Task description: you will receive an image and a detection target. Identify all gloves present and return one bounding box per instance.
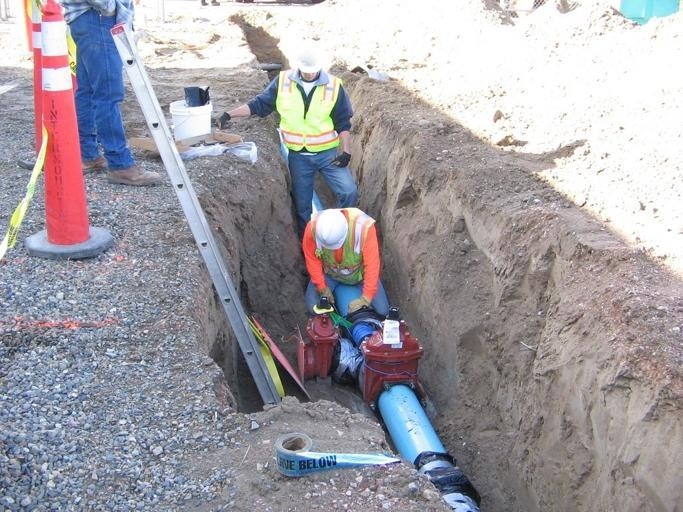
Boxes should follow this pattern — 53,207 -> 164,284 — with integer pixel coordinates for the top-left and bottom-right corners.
334,152 -> 352,171
216,112 -> 231,131
347,297 -> 370,313
318,287 -> 337,303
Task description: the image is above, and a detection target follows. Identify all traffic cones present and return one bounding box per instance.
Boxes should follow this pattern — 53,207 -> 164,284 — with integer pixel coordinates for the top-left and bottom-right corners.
15,0 -> 45,167
10,0 -> 118,263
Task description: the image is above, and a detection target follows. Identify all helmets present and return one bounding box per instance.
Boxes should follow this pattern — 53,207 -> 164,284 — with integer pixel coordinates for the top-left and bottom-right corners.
296,46 -> 326,74
317,208 -> 348,250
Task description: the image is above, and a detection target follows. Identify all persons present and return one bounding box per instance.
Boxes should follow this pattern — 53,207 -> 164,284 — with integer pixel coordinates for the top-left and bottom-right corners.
60,0 -> 161,188
216,43 -> 358,239
302,207 -> 390,317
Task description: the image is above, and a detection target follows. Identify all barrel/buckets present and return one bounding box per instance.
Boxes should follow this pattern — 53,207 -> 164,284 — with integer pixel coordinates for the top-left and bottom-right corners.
169,98 -> 213,142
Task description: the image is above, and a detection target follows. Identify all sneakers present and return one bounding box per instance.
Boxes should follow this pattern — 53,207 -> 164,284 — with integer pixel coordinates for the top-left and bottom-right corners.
108,164 -> 161,187
81,154 -> 110,172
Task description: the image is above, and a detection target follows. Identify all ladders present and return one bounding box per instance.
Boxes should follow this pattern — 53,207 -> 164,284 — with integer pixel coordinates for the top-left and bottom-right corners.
110,21 -> 282,407
